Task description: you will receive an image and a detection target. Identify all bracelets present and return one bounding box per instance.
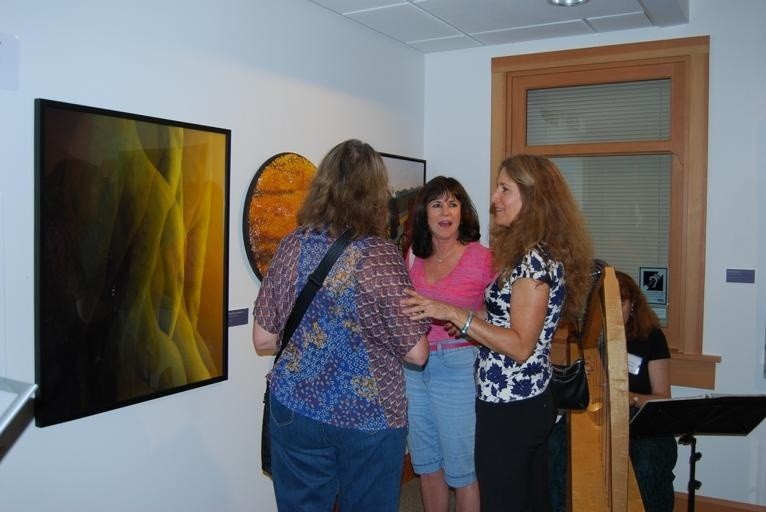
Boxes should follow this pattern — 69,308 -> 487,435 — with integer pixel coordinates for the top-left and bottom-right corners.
630,394 -> 639,409
461,311 -> 473,334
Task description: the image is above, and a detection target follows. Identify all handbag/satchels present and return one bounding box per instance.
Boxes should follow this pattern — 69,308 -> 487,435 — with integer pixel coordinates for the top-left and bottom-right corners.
260,380 -> 271,473
550,358 -> 589,410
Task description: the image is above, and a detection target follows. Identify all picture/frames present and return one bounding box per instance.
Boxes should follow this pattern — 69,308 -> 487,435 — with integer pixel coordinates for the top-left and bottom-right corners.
243,151 -> 318,282
380,153 -> 426,198
34,98 -> 231,428
639,267 -> 667,305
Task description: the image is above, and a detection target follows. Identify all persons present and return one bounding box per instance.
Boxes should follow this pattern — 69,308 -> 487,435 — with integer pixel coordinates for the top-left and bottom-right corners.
599,271 -> 678,512
253,140 -> 433,511
399,154 -> 593,512
403,176 -> 495,512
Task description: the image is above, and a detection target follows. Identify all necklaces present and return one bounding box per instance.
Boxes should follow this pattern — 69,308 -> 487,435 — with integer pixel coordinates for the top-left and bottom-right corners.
435,245 -> 454,263
